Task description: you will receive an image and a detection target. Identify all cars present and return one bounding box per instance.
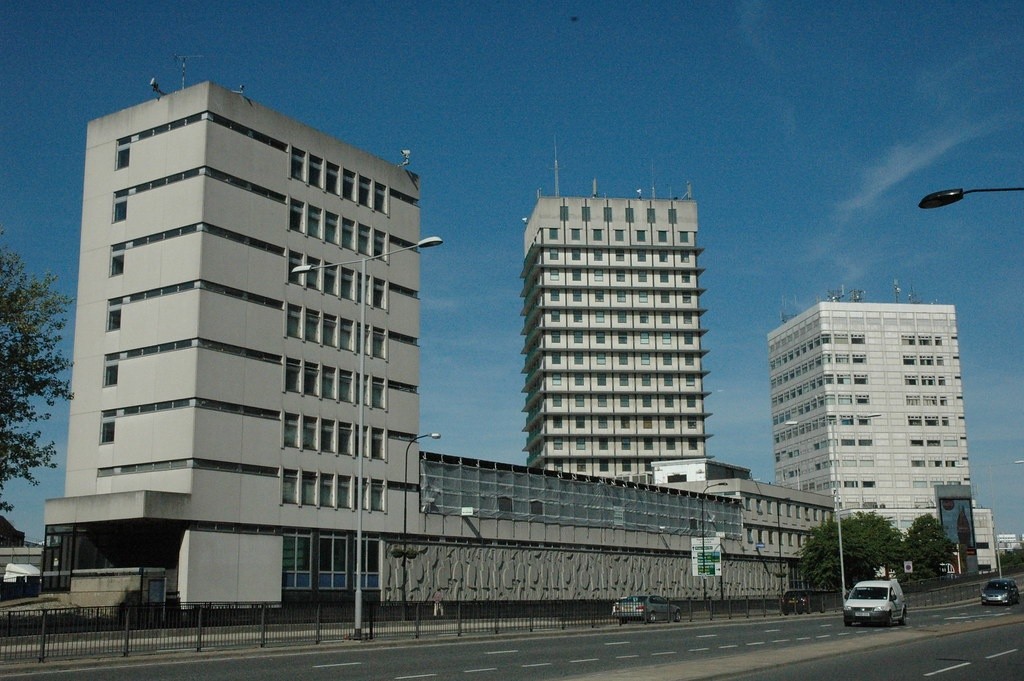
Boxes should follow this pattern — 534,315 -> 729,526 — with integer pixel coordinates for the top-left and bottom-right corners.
843,578 -> 907,627
612,594 -> 682,623
981,578 -> 1020,606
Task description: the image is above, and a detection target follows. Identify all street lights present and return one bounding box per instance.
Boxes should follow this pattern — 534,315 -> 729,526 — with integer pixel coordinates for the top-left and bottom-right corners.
402,433 -> 441,620
777,496 -> 791,596
292,235 -> 441,639
701,481 -> 729,612
954,460 -> 1024,578
785,413 -> 882,608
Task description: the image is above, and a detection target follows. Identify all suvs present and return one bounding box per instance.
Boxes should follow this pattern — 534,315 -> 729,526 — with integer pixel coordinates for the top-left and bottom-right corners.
781,591 -> 825,616
